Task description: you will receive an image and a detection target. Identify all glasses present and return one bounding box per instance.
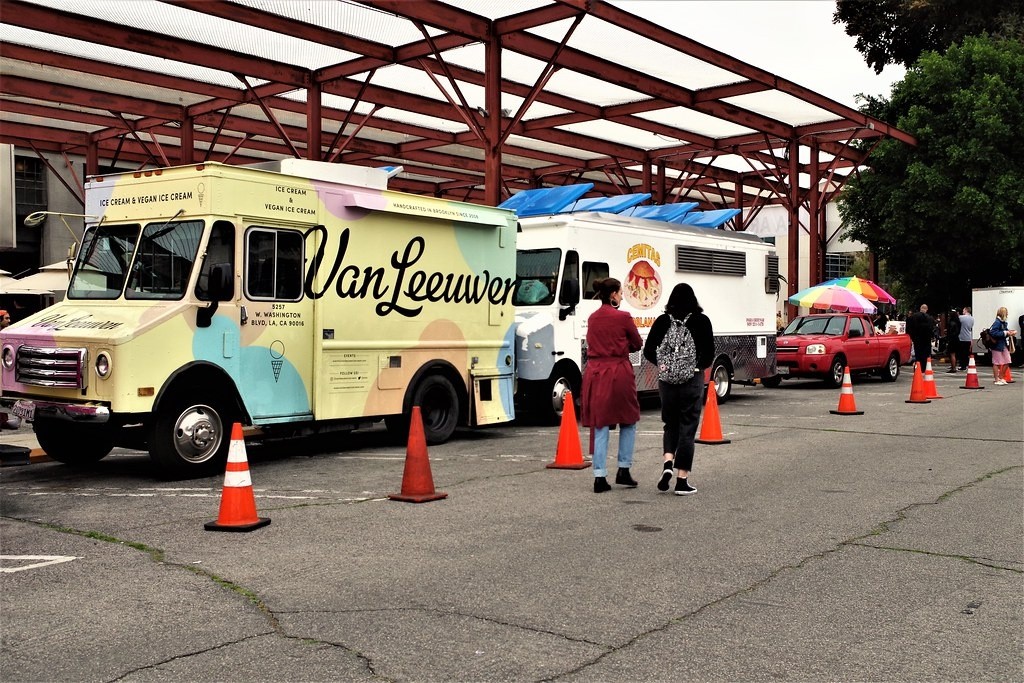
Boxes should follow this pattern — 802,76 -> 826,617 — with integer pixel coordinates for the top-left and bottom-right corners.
5,317 -> 10,319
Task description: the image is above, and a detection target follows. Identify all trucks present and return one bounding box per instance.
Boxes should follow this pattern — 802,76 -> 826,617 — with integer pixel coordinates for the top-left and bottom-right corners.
971,285 -> 1024,368
498,182 -> 781,425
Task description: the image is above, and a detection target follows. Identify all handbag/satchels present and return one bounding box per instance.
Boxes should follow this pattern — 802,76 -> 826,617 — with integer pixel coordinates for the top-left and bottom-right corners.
1009,335 -> 1015,354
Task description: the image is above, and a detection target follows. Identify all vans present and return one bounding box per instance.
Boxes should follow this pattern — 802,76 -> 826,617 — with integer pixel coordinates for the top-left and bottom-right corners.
0,152 -> 519,479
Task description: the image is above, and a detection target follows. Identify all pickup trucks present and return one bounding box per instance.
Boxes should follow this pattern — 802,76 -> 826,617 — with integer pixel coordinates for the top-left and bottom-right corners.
761,312 -> 913,389
780,304 -> 781,305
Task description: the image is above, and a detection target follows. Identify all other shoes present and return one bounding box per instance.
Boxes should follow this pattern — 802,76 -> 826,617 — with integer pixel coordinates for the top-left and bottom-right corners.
615,467 -> 638,486
946,370 -> 956,373
593,477 -> 611,493
958,367 -> 966,370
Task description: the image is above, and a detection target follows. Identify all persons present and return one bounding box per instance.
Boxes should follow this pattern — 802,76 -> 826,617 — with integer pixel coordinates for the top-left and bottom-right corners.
581,277 -> 644,493
911,304 -> 935,372
0,310 -> 11,331
643,282 -> 716,496
872,309 -> 912,331
989,307 -> 1015,386
947,306 -> 974,374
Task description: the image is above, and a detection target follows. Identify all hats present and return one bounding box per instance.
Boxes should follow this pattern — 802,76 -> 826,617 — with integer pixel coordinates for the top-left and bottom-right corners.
0,310 -> 7,316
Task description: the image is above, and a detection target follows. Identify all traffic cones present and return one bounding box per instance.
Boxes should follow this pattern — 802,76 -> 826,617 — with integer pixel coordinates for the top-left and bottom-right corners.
999,364 -> 1017,383
959,355 -> 985,389
545,391 -> 592,471
694,380 -> 732,445
204,421 -> 273,533
904,361 -> 932,403
923,357 -> 944,400
387,405 -> 449,503
828,365 -> 866,417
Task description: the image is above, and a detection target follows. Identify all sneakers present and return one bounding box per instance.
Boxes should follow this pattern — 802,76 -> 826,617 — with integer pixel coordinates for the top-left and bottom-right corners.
674,477 -> 697,495
995,379 -> 1008,386
657,460 -> 674,491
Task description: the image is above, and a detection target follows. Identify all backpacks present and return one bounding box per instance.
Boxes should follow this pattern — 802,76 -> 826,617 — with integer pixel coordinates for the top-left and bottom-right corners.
656,311 -> 697,384
977,319 -> 997,351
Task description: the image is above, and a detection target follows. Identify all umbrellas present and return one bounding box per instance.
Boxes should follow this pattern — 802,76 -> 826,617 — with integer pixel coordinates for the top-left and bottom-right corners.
789,284 -> 878,313
0,256 -> 107,295
815,274 -> 896,305
